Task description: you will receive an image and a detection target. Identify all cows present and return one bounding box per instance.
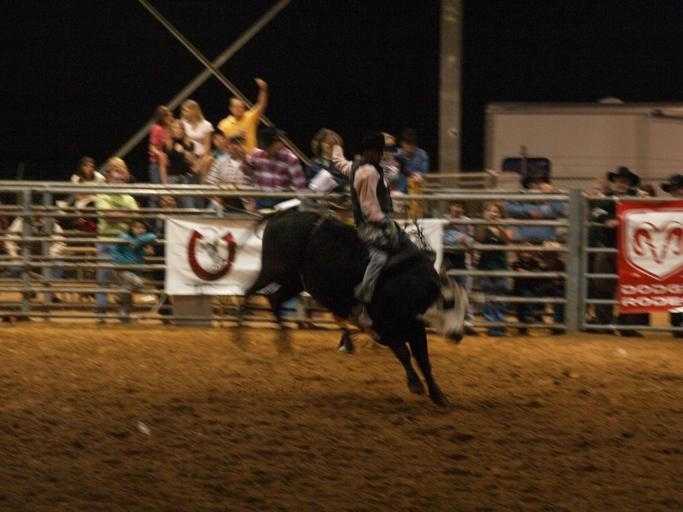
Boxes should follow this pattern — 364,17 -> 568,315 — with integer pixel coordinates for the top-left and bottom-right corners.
233,203 -> 471,410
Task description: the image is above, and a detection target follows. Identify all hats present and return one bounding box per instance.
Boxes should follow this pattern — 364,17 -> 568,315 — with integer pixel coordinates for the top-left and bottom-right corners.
355,129 -> 417,152
522,171 -> 548,188
662,175 -> 683,191
607,166 -> 640,186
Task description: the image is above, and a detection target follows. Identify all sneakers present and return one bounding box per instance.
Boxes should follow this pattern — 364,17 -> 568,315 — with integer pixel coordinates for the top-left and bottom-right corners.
348,305 -> 373,326
